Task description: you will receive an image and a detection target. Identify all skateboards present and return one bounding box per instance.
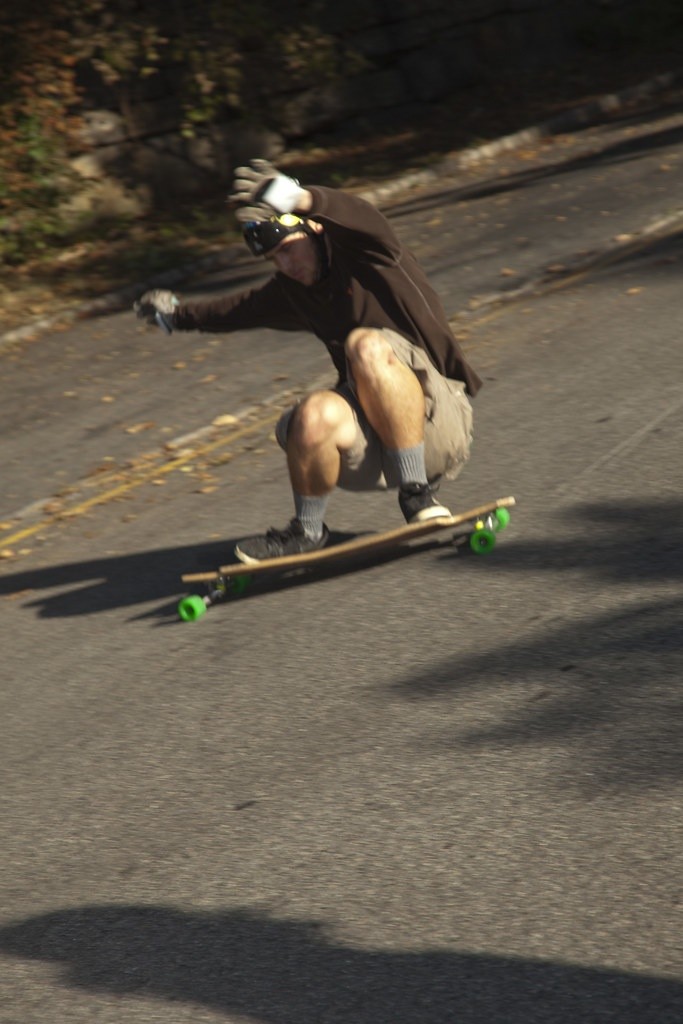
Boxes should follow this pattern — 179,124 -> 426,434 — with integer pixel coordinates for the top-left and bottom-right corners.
175,495 -> 517,619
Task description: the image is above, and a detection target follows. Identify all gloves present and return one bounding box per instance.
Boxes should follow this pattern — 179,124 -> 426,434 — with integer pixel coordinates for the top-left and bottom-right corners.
132,287 -> 178,332
228,158 -> 301,215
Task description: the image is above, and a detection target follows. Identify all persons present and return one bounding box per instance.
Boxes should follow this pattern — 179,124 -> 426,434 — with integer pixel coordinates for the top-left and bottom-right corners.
133,156 -> 481,564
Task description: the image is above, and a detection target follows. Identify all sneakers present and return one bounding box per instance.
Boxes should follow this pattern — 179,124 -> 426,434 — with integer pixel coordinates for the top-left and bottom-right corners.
397,483 -> 451,524
234,518 -> 330,568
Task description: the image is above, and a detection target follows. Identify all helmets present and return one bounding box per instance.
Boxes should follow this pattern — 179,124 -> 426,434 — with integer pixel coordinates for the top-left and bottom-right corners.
241,211 -> 308,256
